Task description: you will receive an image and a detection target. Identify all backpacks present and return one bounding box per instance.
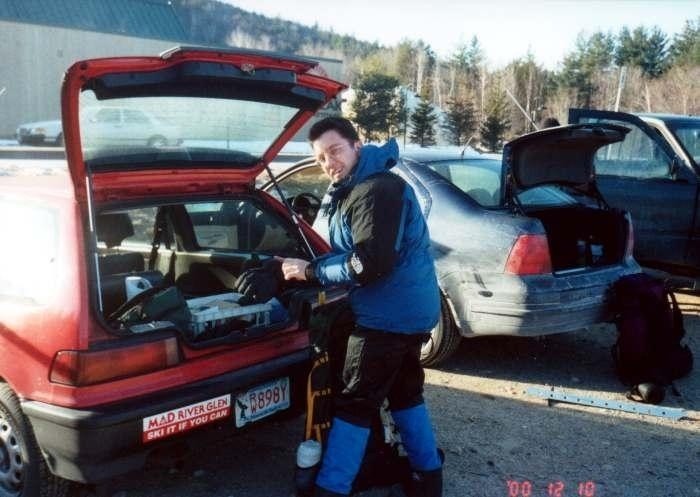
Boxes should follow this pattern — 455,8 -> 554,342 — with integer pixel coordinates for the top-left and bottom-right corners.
605,275 -> 693,383
295,350 -> 413,495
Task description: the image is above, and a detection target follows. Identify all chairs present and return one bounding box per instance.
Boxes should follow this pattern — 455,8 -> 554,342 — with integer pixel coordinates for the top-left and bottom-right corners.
95,214 -> 147,297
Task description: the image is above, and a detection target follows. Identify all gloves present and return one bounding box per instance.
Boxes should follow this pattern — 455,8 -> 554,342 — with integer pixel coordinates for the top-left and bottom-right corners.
234,262 -> 284,303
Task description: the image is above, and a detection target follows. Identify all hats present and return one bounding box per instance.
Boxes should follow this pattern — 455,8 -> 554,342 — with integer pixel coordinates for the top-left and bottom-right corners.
107,275 -> 161,315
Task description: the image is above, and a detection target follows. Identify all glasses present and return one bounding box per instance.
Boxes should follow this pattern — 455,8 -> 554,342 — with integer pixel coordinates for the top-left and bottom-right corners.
315,141 -> 351,168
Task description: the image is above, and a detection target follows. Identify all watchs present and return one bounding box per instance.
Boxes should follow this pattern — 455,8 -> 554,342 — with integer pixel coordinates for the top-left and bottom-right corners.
305,264 -> 317,282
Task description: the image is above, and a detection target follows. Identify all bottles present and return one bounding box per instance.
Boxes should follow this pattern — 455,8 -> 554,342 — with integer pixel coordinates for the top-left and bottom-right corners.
292,439 -> 324,492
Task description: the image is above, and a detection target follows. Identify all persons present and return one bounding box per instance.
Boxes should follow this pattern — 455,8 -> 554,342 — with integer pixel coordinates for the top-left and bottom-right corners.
273,118 -> 442,497
